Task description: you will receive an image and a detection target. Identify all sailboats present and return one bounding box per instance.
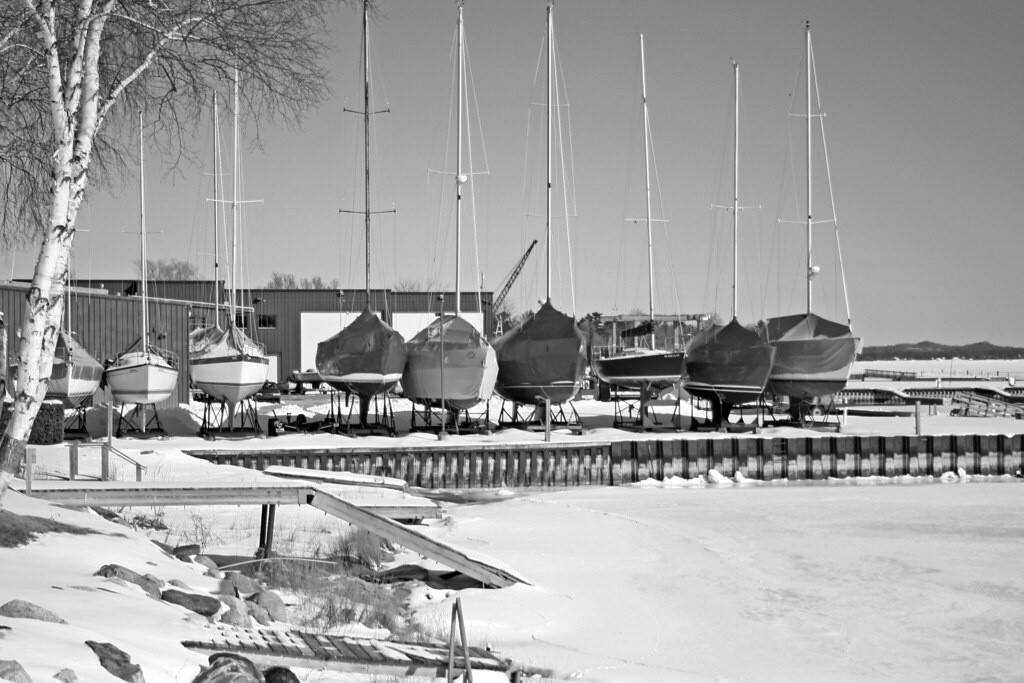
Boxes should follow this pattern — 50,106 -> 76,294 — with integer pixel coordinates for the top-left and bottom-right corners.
311,0 -> 410,392
750,16 -> 864,401
402,0 -> 502,411
99,107 -> 180,405
593,29 -> 688,386
183,57 -> 270,399
483,5 -> 587,407
676,58 -> 779,405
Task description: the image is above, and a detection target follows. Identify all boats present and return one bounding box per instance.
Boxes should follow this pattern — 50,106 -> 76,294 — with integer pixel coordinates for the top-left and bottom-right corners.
6,252 -> 104,405
